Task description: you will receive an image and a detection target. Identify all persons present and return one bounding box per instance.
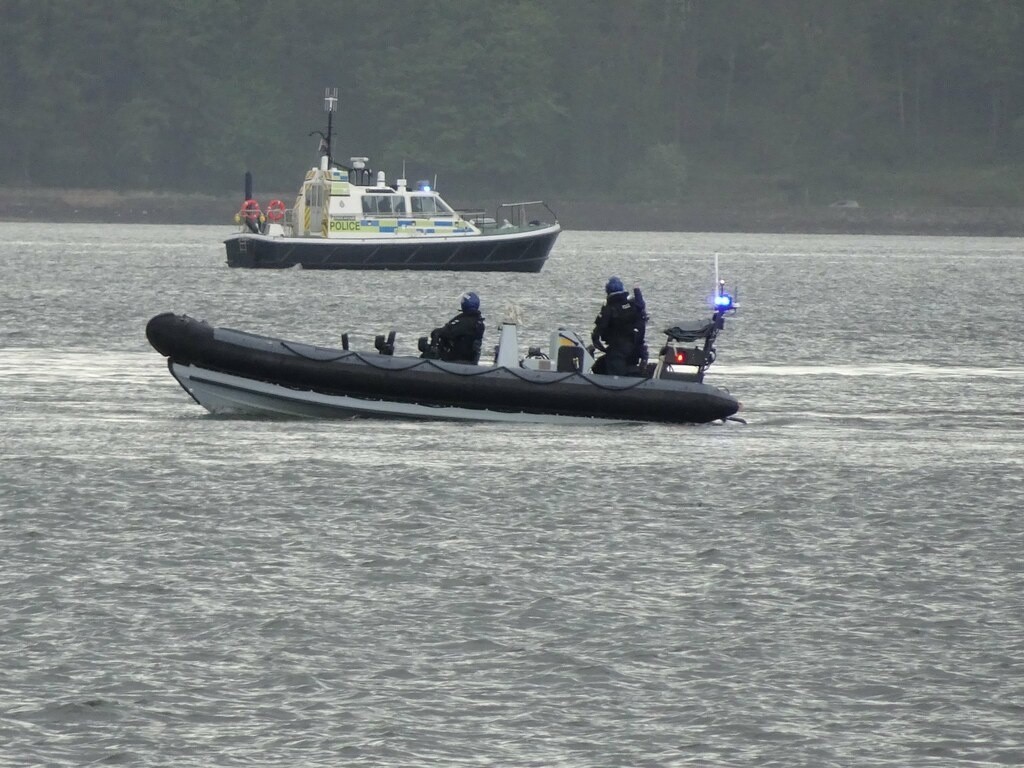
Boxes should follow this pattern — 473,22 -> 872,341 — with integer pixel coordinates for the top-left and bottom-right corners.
430,293 -> 486,364
362,197 -> 419,213
591,276 -> 648,376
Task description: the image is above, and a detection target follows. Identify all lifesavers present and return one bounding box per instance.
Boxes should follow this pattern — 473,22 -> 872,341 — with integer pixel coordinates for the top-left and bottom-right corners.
266,199 -> 287,221
240,199 -> 260,221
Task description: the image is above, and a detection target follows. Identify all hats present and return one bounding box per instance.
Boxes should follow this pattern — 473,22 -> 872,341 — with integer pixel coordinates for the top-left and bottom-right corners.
457,292 -> 480,312
605,276 -> 623,293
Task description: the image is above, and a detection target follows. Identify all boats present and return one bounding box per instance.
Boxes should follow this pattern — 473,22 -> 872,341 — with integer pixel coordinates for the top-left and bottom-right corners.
223,88 -> 562,275
146,253 -> 746,426
